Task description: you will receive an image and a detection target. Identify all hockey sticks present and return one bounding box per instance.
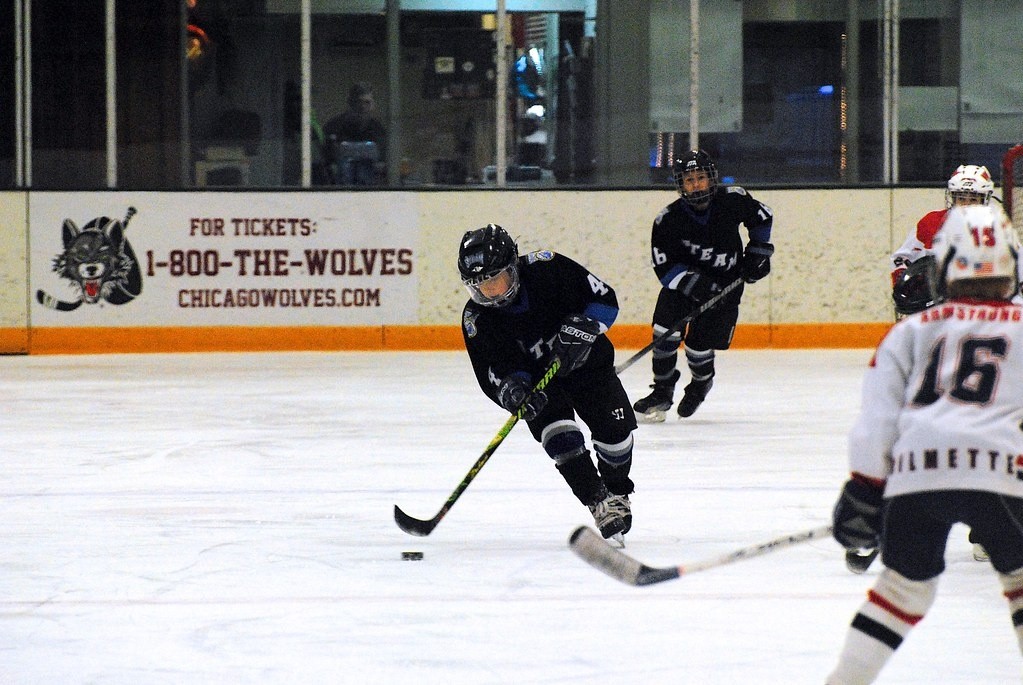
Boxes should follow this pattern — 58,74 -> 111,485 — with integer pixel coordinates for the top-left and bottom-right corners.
393,357 -> 562,538
564,522 -> 834,590
615,277 -> 744,375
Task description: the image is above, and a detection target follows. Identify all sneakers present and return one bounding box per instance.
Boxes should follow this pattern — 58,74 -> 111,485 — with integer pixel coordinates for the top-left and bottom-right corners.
677,378 -> 713,420
587,482 -> 635,549
968,530 -> 992,562
632,385 -> 674,424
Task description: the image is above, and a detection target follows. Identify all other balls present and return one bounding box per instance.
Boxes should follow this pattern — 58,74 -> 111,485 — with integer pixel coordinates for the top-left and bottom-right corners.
401,552 -> 423,561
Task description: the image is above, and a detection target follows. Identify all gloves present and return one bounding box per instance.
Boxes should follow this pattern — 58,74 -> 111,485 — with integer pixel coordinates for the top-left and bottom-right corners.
676,272 -> 722,309
832,471 -> 885,550
741,242 -> 774,284
498,374 -> 547,422
545,316 -> 600,376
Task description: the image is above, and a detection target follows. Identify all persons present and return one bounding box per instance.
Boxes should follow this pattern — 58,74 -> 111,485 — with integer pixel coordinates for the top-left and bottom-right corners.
458,224 -> 640,550
826,204 -> 1022,684
891,165 -> 1023,562
634,150 -> 774,424
322,80 -> 388,190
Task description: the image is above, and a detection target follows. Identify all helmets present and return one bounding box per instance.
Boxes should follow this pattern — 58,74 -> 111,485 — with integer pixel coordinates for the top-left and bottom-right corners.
672,149 -> 719,206
946,165 -> 995,210
931,204 -> 1018,288
457,225 -> 521,307
892,255 -> 947,323
